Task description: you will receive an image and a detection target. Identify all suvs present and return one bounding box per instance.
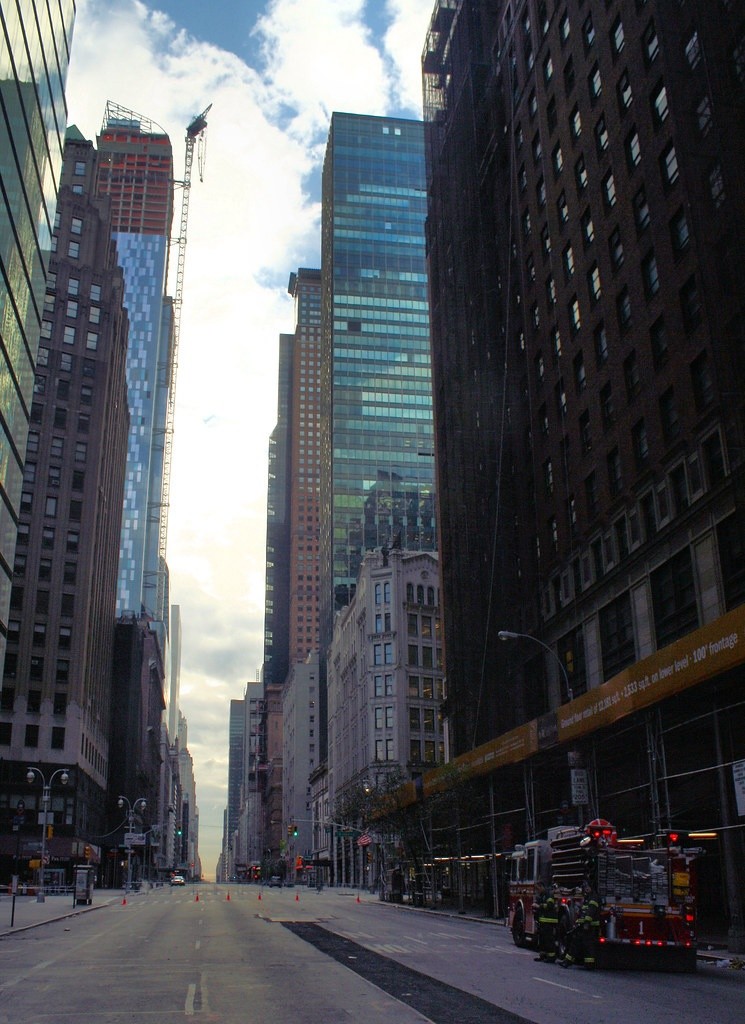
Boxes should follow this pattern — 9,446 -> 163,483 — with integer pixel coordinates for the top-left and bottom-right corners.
269,876 -> 282,888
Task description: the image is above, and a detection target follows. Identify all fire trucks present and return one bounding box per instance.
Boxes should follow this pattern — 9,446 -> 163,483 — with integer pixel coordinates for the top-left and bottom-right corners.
507,817 -> 706,975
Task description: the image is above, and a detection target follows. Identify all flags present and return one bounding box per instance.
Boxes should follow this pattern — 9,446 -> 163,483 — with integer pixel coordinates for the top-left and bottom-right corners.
358,827 -> 373,846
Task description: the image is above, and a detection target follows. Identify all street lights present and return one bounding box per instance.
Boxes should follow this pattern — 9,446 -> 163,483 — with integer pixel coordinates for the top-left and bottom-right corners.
117,795 -> 147,895
27,768 -> 70,904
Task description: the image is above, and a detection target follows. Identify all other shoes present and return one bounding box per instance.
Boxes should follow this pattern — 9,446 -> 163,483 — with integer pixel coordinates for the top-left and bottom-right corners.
534,958 -> 544,961
558,962 -> 567,969
543,959 -> 555,963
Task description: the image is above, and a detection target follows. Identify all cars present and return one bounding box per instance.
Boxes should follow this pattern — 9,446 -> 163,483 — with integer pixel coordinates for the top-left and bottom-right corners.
171,876 -> 185,886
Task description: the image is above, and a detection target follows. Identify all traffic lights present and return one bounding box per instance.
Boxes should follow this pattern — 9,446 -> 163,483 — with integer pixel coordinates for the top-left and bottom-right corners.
293,825 -> 298,836
287,825 -> 293,836
177,825 -> 182,835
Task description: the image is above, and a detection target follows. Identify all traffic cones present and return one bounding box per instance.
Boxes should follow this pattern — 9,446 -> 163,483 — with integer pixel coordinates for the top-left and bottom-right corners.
195,895 -> 199,901
356,897 -> 361,903
294,894 -> 299,901
227,894 -> 230,901
121,899 -> 127,905
258,892 -> 262,900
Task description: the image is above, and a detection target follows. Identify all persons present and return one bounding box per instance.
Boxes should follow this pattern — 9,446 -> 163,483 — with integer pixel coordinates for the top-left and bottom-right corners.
530,881 -> 561,964
556,882 -> 602,970
